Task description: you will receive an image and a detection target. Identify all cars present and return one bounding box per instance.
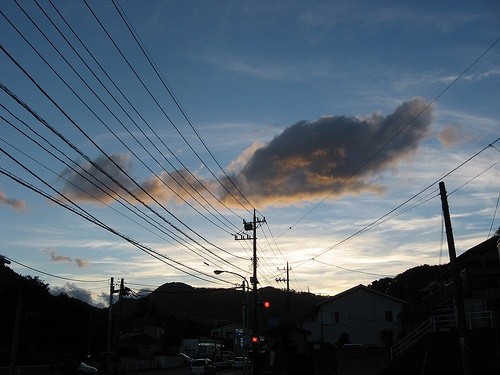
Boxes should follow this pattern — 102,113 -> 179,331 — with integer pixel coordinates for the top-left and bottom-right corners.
188,342 -> 252,375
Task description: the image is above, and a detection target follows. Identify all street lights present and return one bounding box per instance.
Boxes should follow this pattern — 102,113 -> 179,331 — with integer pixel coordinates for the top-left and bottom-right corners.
214,270 -> 249,350
